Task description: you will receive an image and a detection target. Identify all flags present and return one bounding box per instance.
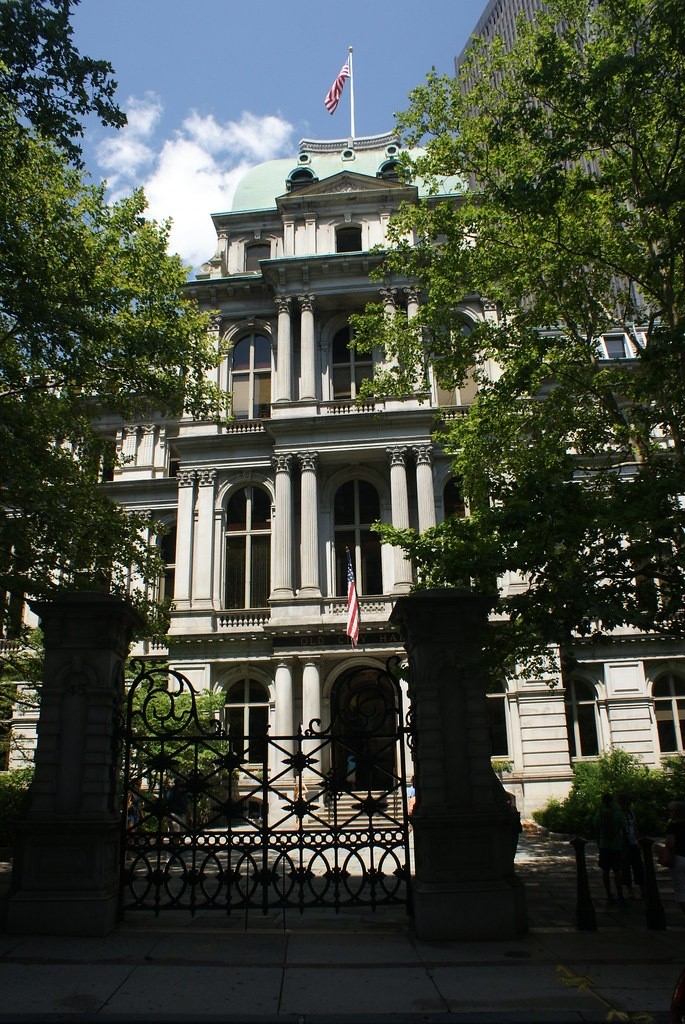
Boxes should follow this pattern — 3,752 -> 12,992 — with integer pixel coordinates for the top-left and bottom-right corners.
346,559 -> 360,648
325,56 -> 350,115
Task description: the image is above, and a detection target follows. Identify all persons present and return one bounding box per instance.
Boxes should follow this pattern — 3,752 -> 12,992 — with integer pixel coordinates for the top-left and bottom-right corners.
408,777 -> 415,833
347,753 -> 356,790
